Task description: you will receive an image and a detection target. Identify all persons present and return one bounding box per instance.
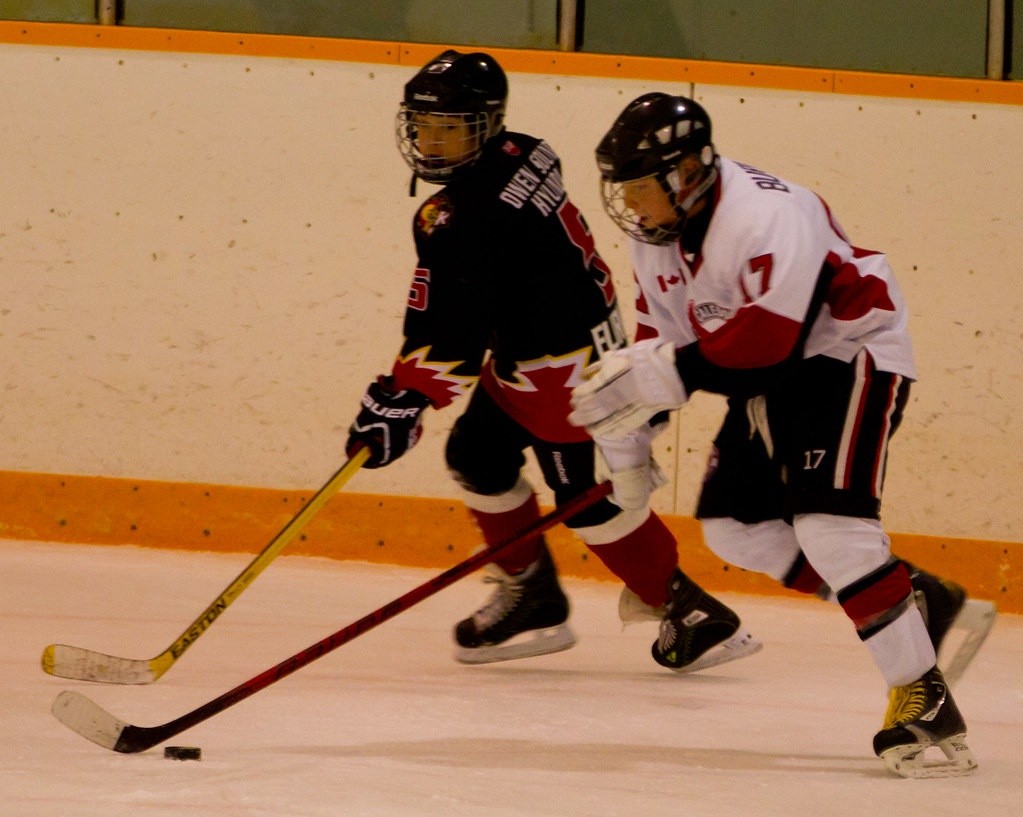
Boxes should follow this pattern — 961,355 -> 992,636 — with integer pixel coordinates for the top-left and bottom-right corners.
344,48 -> 743,671
566,91 -> 985,760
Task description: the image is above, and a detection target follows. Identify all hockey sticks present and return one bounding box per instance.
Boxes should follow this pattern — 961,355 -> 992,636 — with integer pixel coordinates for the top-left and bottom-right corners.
49,477 -> 616,755
40,444 -> 371,685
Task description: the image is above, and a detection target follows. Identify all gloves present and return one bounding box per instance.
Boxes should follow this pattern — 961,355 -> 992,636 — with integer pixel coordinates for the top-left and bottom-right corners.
568,337 -> 688,445
593,442 -> 650,511
344,372 -> 436,470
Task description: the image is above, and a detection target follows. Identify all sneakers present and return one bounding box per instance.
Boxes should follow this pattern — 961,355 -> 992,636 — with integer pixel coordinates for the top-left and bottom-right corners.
451,532 -> 579,665
897,558 -> 998,691
873,663 -> 979,779
618,564 -> 763,674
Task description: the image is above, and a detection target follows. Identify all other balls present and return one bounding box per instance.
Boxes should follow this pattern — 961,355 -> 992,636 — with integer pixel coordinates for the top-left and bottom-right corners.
164,746 -> 200,761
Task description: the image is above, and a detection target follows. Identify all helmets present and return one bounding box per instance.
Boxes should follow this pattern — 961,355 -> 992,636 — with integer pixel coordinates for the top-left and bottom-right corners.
395,51 -> 508,185
595,91 -> 717,247
